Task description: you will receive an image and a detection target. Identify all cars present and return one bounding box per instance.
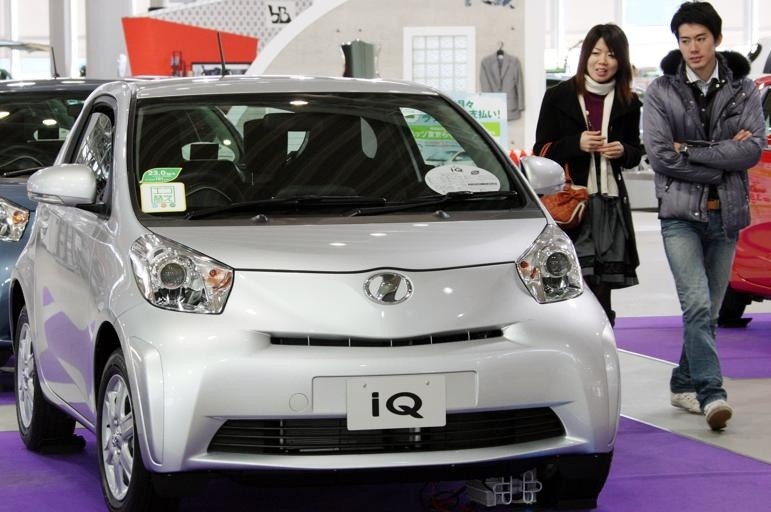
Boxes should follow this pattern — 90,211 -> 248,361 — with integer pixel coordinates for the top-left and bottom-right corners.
541,70 -> 652,181
717,73 -> 771,323
7,70 -> 626,512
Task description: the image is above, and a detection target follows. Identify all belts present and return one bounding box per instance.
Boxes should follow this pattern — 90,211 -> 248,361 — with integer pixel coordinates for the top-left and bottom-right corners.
705,198 -> 720,213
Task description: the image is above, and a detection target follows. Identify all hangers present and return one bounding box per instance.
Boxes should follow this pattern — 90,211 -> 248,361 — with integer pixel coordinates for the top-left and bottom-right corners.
480,41 -> 520,65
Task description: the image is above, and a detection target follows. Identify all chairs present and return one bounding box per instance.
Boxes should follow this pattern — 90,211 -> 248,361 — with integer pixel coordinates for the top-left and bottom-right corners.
143,111 -> 419,203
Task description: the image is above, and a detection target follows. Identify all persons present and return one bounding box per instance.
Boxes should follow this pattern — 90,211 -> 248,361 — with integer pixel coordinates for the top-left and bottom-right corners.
641,0 -> 769,430
533,22 -> 645,331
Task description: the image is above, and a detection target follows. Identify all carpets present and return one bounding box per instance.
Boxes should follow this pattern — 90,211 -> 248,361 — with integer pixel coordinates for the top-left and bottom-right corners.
613,313 -> 771,380
1,413 -> 771,512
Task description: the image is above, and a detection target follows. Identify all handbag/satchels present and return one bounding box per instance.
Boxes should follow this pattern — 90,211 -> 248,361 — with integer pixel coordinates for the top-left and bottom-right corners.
535,143 -> 591,229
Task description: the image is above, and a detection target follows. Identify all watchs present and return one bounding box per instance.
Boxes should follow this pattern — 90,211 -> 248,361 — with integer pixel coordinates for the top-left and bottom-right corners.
680,139 -> 688,157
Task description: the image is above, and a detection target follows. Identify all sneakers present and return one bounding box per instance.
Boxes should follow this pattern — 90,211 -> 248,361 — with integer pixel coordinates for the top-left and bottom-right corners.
704,400 -> 733,429
670,391 -> 701,415
